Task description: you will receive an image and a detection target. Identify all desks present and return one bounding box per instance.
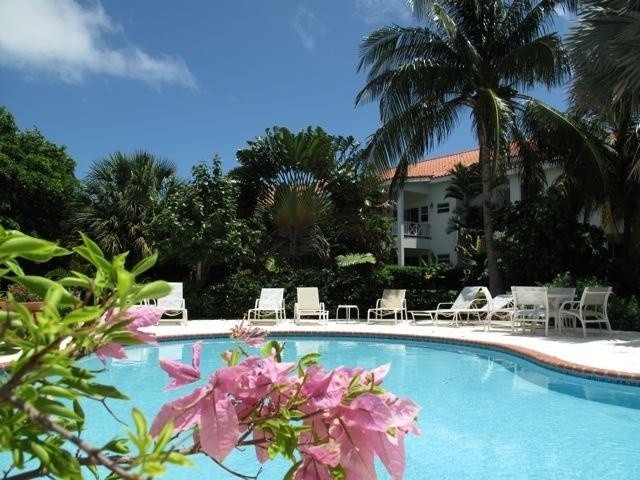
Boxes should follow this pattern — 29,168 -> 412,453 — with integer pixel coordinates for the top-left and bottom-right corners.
336,304 -> 360,324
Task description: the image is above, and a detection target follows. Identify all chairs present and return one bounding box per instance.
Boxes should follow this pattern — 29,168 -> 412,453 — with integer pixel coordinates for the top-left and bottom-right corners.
293,287 -> 329,326
366,285 -> 613,340
248,288 -> 286,326
140,281 -> 187,327
